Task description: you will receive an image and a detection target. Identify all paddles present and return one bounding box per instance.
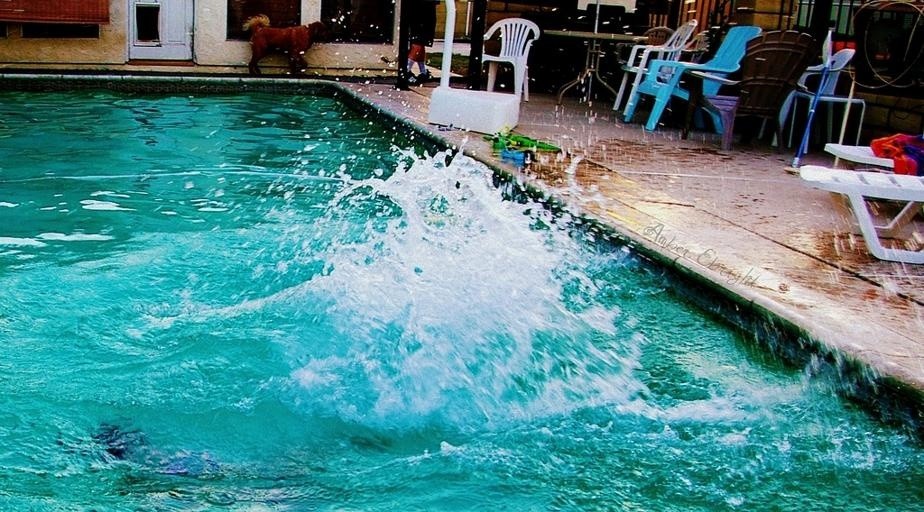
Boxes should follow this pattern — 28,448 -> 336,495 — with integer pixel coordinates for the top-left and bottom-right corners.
793,30 -> 831,168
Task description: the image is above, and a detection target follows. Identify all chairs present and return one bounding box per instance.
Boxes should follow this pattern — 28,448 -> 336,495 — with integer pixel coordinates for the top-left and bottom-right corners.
800,165 -> 924,264
481,18 -> 540,104
824,143 -> 894,173
613,18 -> 866,154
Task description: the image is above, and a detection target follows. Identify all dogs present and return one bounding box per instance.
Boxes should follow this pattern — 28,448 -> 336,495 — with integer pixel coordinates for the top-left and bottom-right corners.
243,14 -> 328,75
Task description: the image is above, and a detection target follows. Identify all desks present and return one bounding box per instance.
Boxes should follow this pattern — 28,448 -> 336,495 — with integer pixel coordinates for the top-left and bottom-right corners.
544,30 -> 649,110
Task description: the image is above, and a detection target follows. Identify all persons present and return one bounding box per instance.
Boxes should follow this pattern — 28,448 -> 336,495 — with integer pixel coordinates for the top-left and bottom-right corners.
399,0 -> 444,86
